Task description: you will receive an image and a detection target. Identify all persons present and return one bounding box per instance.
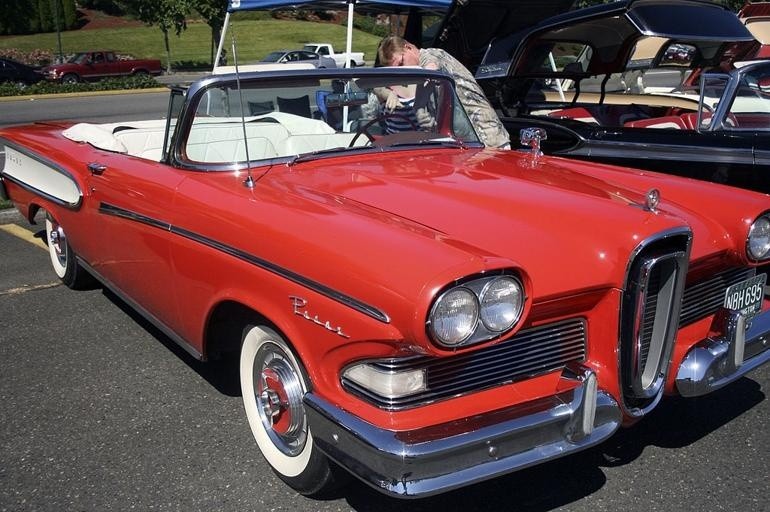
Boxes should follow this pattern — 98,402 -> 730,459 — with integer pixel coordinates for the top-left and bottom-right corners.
372,35 -> 511,150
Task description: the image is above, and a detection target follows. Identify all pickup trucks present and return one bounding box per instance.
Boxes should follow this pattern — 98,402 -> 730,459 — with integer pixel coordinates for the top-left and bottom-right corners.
42,47 -> 165,83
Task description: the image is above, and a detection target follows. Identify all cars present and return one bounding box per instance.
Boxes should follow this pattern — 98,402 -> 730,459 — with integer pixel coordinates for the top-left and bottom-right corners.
0,54 -> 50,89
0,52 -> 770,507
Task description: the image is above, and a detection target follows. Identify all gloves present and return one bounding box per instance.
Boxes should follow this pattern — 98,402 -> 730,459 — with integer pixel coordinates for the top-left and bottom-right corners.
373,87 -> 405,114
413,80 -> 438,128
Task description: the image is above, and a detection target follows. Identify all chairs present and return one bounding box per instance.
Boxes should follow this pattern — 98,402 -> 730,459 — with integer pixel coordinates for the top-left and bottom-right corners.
548,107 -> 600,127
680,111 -> 740,131
624,115 -> 689,131
114,120 -> 374,163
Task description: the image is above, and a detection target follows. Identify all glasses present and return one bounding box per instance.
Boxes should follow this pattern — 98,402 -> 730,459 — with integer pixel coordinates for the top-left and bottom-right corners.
398,54 -> 404,66
